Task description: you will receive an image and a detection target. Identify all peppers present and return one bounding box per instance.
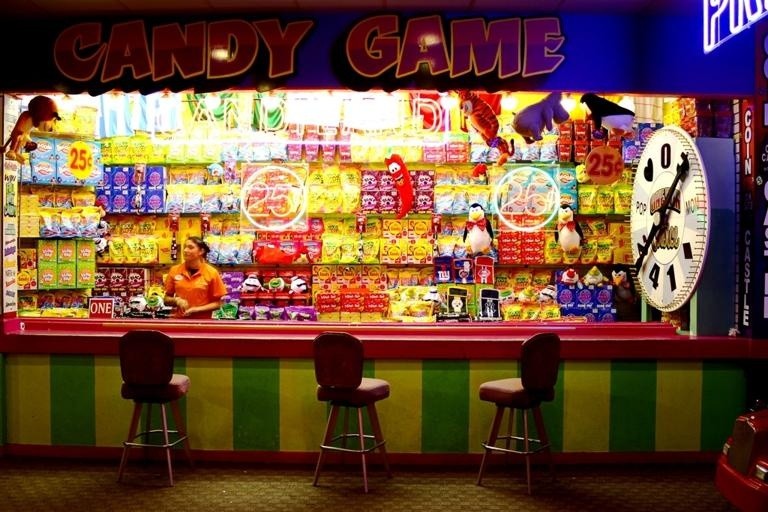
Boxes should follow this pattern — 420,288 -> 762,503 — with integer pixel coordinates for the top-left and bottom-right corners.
384,148 -> 413,219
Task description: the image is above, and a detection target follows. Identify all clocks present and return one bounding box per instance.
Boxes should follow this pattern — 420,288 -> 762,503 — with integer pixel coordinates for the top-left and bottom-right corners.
628,124 -> 713,317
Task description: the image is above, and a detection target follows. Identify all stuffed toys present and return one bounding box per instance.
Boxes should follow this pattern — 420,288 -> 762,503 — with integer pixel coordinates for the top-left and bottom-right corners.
462,202 -> 495,257
454,89 -> 516,176
579,91 -> 637,142
384,148 -> 417,221
553,204 -> 590,256
513,91 -> 571,145
0,95 -> 63,165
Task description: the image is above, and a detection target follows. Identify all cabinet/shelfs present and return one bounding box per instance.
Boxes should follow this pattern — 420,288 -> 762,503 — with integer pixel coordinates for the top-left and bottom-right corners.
17,156 -> 632,289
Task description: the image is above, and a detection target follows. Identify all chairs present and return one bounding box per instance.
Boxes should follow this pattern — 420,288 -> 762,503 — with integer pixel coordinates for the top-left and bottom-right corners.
474,331 -> 564,495
112,329 -> 194,488
310,329 -> 394,494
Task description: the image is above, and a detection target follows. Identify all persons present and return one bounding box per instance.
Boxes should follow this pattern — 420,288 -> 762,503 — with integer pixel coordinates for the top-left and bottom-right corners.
164,235 -> 229,319
